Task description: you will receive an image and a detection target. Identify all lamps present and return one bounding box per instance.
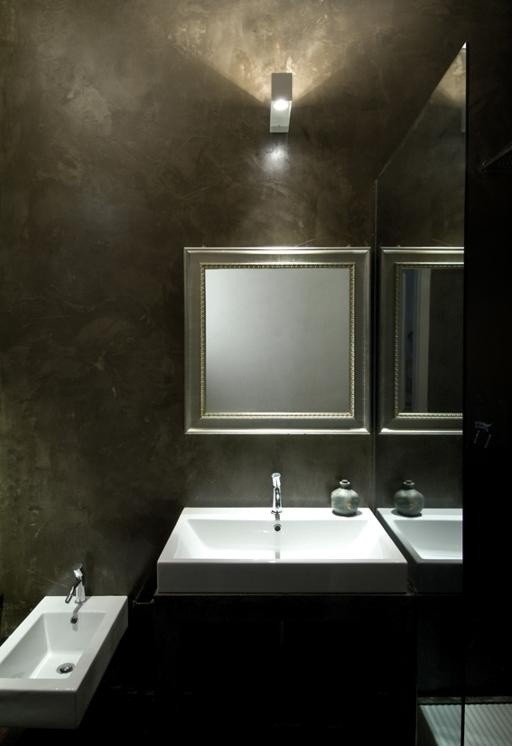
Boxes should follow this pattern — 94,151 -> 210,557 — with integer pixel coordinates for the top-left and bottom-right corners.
269,72 -> 296,110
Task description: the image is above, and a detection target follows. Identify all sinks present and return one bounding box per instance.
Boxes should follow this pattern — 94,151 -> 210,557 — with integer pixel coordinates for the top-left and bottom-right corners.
376,504 -> 463,592
156,506 -> 411,596
0,593 -> 130,726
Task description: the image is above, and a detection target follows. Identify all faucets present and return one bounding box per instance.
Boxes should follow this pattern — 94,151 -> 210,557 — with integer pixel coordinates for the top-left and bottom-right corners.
272,470 -> 282,515
64,566 -> 86,608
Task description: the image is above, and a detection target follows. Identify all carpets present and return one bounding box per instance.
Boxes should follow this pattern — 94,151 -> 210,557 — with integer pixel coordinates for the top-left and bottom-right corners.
419,703 -> 512,746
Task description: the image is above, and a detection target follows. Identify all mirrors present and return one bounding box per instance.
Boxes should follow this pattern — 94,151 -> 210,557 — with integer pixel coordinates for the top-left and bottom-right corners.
377,243 -> 470,437
183,245 -> 372,436
376,33 -> 467,572
